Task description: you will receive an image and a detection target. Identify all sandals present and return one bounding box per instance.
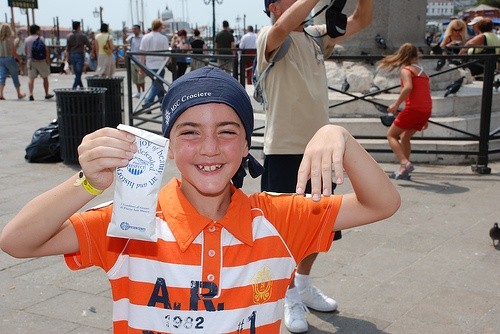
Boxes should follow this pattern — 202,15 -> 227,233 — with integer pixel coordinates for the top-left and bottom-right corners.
387,159 -> 415,181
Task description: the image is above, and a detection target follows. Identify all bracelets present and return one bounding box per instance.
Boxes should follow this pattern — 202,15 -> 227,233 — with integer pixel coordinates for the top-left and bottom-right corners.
74,172 -> 104,195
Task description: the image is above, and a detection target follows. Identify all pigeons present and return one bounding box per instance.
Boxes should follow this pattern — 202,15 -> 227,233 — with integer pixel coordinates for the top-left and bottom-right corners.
493,78 -> 500,92
436,58 -> 446,71
377,33 -> 387,50
444,76 -> 466,97
488,222 -> 500,246
369,85 -> 380,96
341,78 -> 349,92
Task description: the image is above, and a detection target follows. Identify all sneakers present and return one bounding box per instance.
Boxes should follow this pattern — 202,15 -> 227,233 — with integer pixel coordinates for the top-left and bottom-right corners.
283,295 -> 310,333
298,283 -> 338,313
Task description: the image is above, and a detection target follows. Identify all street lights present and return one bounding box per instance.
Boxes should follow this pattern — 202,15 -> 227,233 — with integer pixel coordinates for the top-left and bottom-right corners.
203,0 -> 223,49
91,4 -> 104,28
235,13 -> 246,34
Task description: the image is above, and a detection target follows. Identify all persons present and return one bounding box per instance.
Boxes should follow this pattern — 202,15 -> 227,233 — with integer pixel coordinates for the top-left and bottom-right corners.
95,23 -> 115,77
14,31 -> 25,75
438,10 -> 500,81
171,29 -> 193,80
0,66 -> 401,334
380,43 -> 433,180
123,25 -> 145,99
191,29 -> 208,71
60,21 -> 94,89
24,25 -> 54,100
0,24 -> 26,99
256,0 -> 375,333
239,26 -> 257,84
215,21 -> 236,75
139,20 -> 171,114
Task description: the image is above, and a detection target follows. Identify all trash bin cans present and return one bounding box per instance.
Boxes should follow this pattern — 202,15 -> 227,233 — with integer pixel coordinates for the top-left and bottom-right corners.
53,87 -> 107,165
84,75 -> 125,128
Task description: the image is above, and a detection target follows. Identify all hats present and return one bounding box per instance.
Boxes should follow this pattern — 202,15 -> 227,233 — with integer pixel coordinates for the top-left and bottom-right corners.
467,15 -> 484,25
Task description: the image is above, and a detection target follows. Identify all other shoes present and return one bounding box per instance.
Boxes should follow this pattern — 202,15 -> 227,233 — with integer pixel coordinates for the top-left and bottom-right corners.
29,96 -> 34,100
141,103 -> 151,114
45,94 -> 54,99
18,93 -> 26,99
133,95 -> 141,99
0,96 -> 6,100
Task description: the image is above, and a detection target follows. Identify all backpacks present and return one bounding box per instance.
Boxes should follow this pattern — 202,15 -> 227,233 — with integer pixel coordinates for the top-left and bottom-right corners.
31,35 -> 47,60
250,26 -> 326,103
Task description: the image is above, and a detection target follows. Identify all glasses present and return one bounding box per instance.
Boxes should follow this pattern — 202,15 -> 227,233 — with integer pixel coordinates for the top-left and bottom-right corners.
453,27 -> 463,31
